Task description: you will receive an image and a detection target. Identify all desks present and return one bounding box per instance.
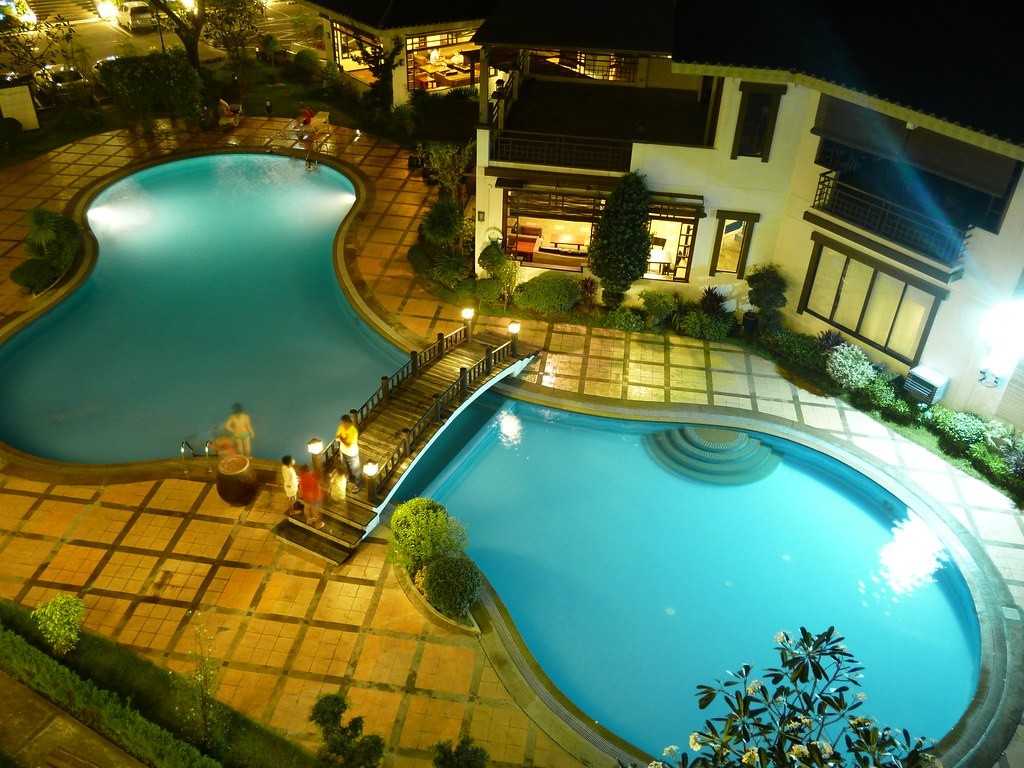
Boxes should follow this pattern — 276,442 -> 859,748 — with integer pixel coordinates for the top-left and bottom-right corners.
435,68 -> 480,88
646,251 -> 672,274
417,76 -> 435,89
550,232 -> 586,249
418,63 -> 448,88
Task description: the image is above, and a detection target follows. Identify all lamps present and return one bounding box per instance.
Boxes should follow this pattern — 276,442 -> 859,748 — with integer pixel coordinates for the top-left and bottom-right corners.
308,437 -> 324,454
508,320 -> 522,334
266,98 -> 271,106
363,459 -> 379,477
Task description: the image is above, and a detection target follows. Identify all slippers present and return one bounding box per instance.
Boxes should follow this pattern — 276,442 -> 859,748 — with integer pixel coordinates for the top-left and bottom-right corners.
306,517 -> 326,530
351,486 -> 360,493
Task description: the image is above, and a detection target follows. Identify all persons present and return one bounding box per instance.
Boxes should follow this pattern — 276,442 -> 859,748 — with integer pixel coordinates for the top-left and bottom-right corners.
216,98 -> 238,117
216,433 -> 236,457
281,455 -> 302,515
335,414 -> 362,494
299,462 -> 326,529
293,102 -> 311,129
329,462 -> 349,501
225,403 -> 255,461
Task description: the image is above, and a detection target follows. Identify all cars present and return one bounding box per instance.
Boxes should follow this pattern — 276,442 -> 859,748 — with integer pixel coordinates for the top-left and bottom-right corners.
0,64 -> 16,82
93,55 -> 121,95
116,1 -> 158,32
33,63 -> 92,112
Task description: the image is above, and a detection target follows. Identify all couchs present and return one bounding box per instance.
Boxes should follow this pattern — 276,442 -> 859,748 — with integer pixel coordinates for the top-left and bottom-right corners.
532,247 -> 589,266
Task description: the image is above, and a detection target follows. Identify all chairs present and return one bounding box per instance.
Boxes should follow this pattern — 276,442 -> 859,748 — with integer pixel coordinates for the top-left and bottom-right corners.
219,104 -> 244,127
648,237 -> 667,272
508,221 -> 542,259
300,111 -> 330,135
